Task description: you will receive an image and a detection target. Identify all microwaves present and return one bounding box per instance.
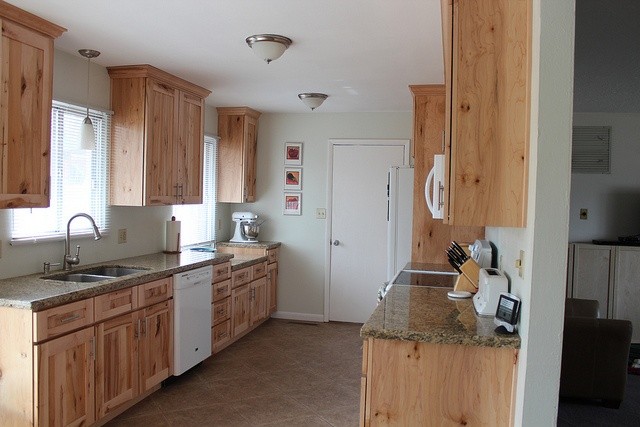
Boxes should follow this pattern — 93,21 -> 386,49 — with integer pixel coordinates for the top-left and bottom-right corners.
424,154 -> 446,221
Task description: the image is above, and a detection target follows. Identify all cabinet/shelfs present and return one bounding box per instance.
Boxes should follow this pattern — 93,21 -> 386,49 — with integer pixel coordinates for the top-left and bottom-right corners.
442,1 -> 533,229
359,263 -> 521,427
215,106 -> 260,205
0,0 -> 69,209
33,285 -> 136,427
567,242 -> 640,344
232,255 -> 267,343
408,263 -> 481,335
211,253 -> 234,354
408,84 -> 492,263
267,247 -> 279,321
135,275 -> 174,404
106,64 -> 212,207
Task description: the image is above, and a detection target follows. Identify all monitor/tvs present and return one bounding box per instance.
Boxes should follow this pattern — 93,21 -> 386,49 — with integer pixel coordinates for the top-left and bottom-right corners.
496,292 -> 521,323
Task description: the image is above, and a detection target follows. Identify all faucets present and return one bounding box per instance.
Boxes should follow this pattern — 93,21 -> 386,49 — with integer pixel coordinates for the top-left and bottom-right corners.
64,212 -> 102,271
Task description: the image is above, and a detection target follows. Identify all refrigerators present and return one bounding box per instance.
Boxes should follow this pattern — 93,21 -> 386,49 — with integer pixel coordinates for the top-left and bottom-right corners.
386,165 -> 414,282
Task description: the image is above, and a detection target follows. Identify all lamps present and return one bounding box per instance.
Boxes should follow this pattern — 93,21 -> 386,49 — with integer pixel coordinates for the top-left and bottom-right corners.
298,92 -> 328,111
78,115 -> 101,151
246,35 -> 292,65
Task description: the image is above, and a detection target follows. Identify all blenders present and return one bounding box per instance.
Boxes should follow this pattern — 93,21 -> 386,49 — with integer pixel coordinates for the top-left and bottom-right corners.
228,212 -> 265,243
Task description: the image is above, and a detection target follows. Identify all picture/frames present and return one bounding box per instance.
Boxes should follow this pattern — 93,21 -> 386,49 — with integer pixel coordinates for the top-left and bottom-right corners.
283,141 -> 303,166
283,167 -> 302,190
282,192 -> 302,215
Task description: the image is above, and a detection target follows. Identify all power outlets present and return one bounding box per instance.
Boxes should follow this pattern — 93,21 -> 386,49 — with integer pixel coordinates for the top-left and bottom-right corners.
117,229 -> 128,244
316,208 -> 326,219
580,208 -> 587,220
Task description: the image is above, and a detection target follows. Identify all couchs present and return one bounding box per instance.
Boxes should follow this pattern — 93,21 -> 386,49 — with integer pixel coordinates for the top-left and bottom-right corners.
560,298 -> 633,409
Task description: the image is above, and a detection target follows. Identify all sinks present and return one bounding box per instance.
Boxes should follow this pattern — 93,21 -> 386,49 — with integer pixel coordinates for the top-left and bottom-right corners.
40,273 -> 111,282
72,268 -> 147,277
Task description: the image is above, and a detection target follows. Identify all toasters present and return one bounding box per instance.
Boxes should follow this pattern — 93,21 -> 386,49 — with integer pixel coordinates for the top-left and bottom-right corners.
472,268 -> 508,319
